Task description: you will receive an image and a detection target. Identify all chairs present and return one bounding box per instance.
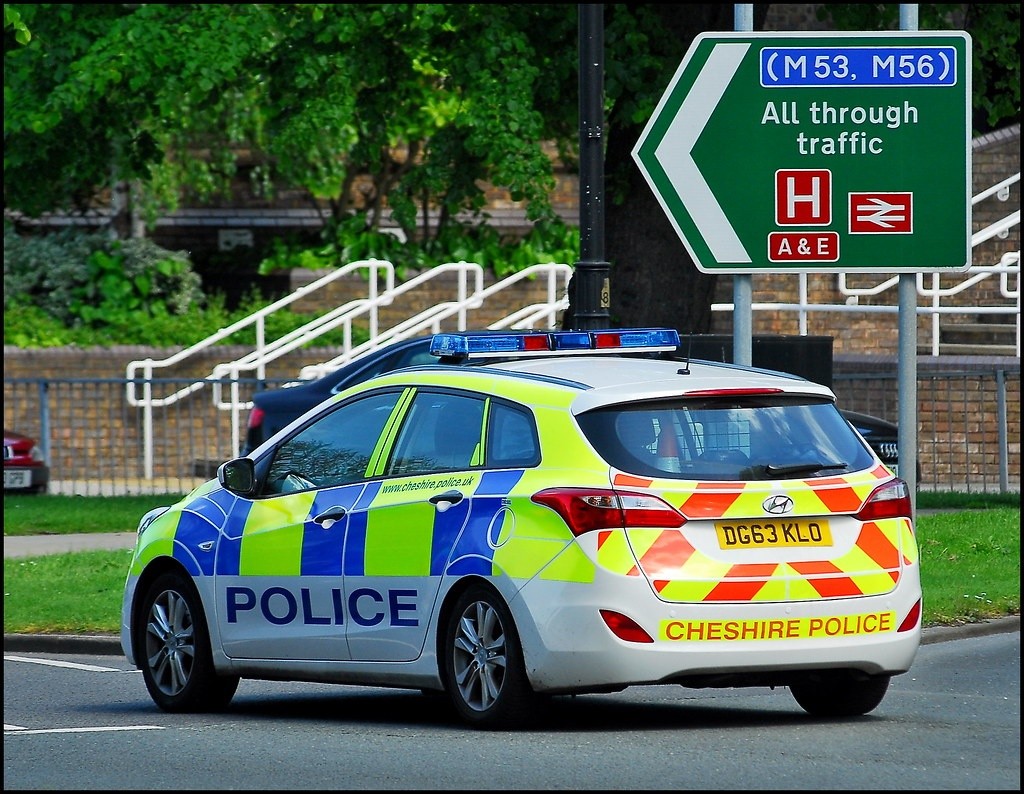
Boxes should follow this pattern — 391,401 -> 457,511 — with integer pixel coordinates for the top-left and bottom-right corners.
403,399 -> 746,475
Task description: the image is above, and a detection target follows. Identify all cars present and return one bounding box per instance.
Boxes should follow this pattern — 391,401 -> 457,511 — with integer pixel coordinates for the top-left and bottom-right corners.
119,327 -> 924,731
240,332 -> 922,491
3,430 -> 49,495
